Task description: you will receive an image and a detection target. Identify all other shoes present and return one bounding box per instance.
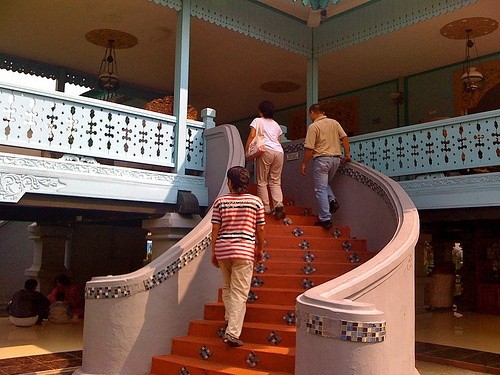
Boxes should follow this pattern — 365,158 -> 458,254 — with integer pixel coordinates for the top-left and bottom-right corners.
274,205 -> 286,220
329,200 -> 340,214
219,326 -> 244,346
314,220 -> 332,228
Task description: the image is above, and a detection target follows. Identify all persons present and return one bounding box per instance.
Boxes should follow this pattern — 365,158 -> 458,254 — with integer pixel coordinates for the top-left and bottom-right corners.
300,105 -> 350,227
8,279 -> 50,326
47,275 -> 80,322
244,101 -> 286,219
212,166 -> 265,345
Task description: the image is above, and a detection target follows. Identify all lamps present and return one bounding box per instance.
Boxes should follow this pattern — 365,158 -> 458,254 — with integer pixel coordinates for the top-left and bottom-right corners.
97,39 -> 120,93
461,29 -> 484,92
390,91 -> 403,106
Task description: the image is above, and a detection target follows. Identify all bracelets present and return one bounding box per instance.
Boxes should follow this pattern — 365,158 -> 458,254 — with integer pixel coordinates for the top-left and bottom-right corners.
345,154 -> 350,156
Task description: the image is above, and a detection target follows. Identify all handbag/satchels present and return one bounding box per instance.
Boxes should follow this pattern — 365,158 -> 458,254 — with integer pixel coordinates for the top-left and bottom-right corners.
244,117 -> 266,162
